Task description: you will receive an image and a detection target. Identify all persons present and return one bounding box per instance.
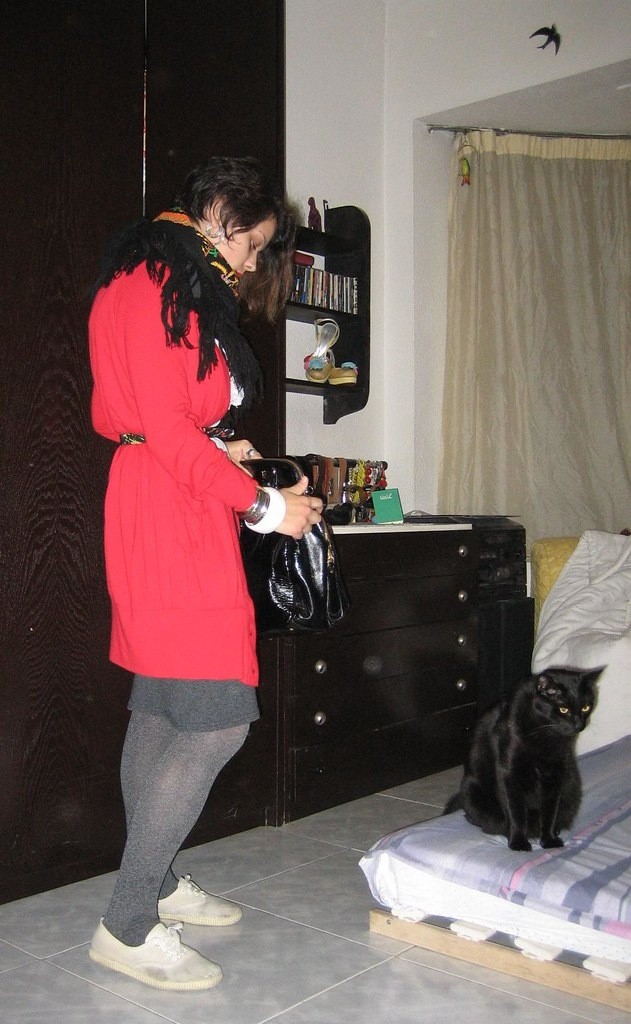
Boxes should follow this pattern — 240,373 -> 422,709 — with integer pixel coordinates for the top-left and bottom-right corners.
86,153 -> 325,990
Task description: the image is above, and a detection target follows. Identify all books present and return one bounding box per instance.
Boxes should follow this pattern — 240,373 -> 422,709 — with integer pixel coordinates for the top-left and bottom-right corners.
371,489 -> 403,524
285,252 -> 358,315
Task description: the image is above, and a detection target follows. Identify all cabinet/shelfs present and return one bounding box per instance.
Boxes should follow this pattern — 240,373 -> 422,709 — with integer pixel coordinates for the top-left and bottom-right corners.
281,516 -> 535,823
284,204 -> 371,424
0,0 -> 281,900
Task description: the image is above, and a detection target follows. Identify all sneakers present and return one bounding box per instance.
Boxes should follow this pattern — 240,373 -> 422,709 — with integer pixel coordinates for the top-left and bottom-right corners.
88,917 -> 224,992
157,874 -> 243,926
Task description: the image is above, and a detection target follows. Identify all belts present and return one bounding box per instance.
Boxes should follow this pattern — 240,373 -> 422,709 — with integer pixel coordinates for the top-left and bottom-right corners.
119,427 -> 235,446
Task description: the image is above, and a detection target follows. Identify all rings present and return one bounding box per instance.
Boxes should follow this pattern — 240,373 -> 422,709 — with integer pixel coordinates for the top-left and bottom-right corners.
247,449 -> 254,455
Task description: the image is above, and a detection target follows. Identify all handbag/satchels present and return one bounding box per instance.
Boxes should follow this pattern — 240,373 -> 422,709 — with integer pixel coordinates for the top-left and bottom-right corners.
236,458 -> 350,633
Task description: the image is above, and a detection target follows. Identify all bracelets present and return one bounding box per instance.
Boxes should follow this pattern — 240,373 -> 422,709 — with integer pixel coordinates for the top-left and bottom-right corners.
239,485 -> 287,534
210,437 -> 229,454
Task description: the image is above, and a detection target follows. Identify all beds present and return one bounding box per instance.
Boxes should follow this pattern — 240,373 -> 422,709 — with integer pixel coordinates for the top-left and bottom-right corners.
358,732 -> 631,1014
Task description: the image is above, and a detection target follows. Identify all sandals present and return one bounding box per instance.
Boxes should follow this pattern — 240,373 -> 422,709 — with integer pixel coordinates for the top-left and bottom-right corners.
304,319 -> 358,385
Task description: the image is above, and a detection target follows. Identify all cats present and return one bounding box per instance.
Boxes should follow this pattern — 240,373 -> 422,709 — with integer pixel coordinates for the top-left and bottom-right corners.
442,664 -> 606,852
